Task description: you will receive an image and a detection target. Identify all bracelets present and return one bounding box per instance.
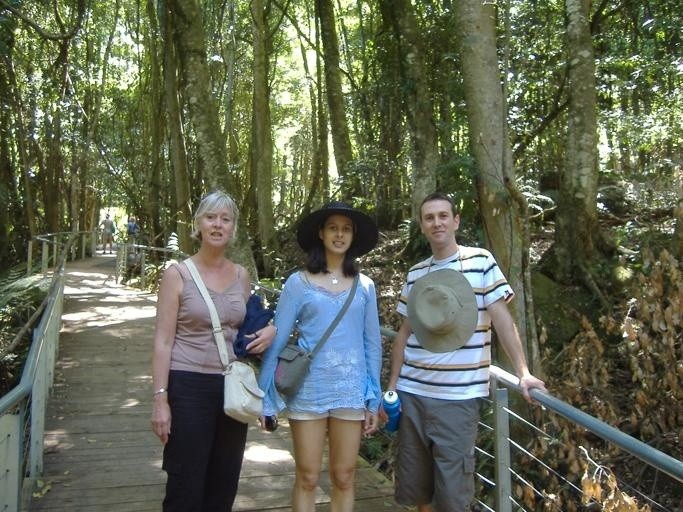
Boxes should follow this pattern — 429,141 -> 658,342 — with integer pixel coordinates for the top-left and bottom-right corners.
153,389 -> 168,396
276,328 -> 278,334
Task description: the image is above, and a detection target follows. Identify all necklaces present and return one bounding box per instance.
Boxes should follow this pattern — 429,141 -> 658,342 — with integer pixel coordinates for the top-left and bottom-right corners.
328,272 -> 342,284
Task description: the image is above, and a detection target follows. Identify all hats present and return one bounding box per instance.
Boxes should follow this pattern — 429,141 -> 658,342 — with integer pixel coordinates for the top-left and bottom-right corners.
297,203 -> 379,257
407,268 -> 479,352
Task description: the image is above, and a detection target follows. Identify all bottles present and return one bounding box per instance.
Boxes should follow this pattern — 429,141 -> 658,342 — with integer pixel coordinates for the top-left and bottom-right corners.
382,390 -> 401,432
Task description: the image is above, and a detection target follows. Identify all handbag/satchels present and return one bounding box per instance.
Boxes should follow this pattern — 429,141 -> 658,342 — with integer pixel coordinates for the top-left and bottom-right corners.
274,343 -> 313,395
223,361 -> 266,425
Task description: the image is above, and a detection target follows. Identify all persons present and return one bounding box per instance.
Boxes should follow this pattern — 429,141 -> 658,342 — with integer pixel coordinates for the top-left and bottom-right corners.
378,196 -> 549,512
151,191 -> 277,512
126,217 -> 140,240
255,201 -> 382,512
96,214 -> 114,254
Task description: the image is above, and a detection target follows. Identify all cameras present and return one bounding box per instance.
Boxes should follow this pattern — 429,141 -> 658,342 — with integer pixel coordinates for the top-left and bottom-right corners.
265,415 -> 279,431
